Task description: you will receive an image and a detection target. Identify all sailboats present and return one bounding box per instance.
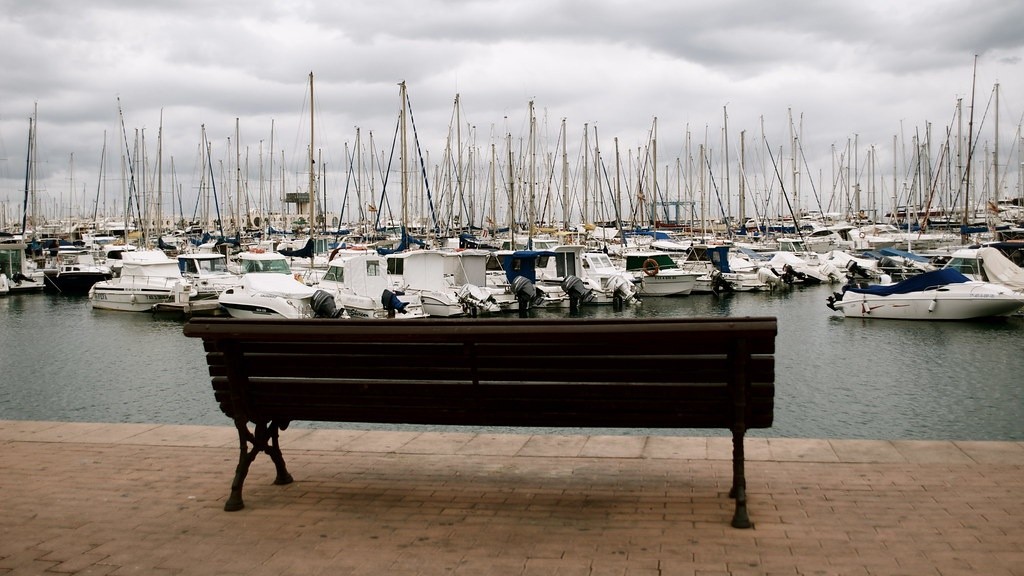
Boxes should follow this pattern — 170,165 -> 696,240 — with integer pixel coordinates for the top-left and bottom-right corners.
0,54 -> 1023,328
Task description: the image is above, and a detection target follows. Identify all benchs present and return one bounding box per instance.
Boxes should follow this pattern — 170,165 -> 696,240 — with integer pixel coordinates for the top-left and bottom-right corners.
183,314 -> 778,532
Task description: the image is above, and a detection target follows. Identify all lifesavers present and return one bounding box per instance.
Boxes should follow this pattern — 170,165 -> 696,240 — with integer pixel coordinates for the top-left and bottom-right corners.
643,259 -> 659,276
859,232 -> 866,238
